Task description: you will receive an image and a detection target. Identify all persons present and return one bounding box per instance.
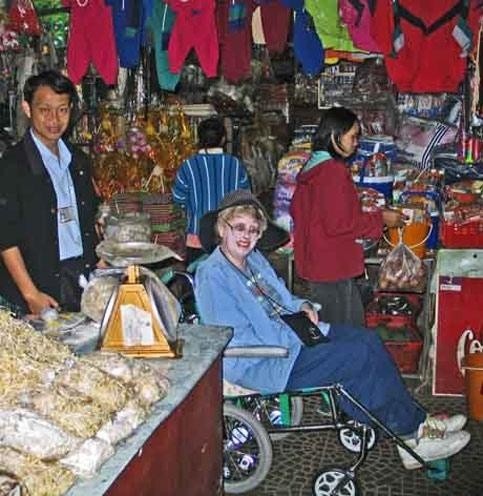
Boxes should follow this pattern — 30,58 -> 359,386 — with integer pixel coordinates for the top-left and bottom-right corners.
288,106 -> 407,329
0,70 -> 103,315
171,118 -> 251,266
193,191 -> 472,470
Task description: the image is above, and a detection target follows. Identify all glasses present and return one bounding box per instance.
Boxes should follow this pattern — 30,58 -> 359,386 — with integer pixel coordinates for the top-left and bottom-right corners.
222,219 -> 261,238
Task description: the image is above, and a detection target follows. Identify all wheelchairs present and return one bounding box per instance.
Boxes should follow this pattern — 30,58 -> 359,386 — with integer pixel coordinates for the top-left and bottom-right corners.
160,252 -> 450,496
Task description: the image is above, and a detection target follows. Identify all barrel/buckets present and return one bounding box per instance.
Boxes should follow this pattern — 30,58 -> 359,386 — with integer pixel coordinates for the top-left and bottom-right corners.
354,153 -> 395,205
462,354 -> 483,422
381,214 -> 434,257
392,191 -> 440,250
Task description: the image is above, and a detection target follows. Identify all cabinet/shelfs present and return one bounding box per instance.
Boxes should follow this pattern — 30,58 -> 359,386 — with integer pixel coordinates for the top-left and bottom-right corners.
105,355 -> 223,491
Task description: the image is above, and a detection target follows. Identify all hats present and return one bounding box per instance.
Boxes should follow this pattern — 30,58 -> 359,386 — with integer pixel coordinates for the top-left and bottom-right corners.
199,189 -> 291,254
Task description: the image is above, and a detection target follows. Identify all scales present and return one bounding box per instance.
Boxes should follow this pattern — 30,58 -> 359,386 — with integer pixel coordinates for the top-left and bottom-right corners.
95,241 -> 185,359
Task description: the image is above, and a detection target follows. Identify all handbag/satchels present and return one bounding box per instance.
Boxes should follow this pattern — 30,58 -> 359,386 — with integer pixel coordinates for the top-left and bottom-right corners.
279,310 -> 330,347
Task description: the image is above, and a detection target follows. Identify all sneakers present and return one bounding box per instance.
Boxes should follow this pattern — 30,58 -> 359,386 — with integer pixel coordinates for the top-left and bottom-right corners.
396,413 -> 471,470
